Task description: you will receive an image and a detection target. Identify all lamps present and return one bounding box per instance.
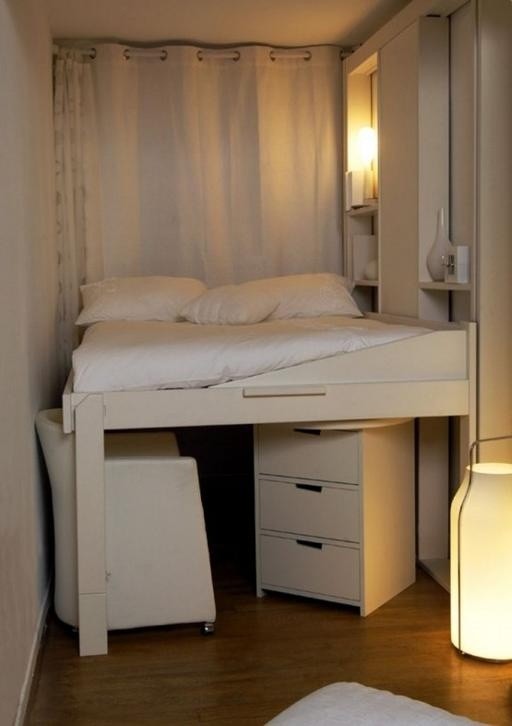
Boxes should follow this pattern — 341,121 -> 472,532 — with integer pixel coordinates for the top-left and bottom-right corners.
353,125 -> 379,201
442,433 -> 512,669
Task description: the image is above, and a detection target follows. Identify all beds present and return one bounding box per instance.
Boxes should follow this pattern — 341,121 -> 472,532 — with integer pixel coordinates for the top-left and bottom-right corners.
58,306 -> 481,659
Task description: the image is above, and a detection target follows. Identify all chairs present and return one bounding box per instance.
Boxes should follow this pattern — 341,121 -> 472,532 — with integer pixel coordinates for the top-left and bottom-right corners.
31,405 -> 219,638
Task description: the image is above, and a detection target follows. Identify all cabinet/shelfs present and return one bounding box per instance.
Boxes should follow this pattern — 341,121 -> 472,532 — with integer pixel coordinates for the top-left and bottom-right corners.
342,194 -> 388,290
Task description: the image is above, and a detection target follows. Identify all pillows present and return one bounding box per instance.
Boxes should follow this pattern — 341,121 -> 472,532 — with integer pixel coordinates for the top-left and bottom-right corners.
242,268 -> 366,328
183,284 -> 284,326
74,273 -> 209,327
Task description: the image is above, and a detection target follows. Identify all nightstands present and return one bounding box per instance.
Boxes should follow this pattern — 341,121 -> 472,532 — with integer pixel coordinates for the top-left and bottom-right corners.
247,414 -> 423,617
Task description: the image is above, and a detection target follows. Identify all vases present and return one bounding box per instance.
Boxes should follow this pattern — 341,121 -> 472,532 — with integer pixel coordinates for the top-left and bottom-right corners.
427,207 -> 458,282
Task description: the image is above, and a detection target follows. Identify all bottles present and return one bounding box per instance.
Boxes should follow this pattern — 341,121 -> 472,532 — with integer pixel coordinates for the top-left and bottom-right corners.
427,206 -> 453,284
